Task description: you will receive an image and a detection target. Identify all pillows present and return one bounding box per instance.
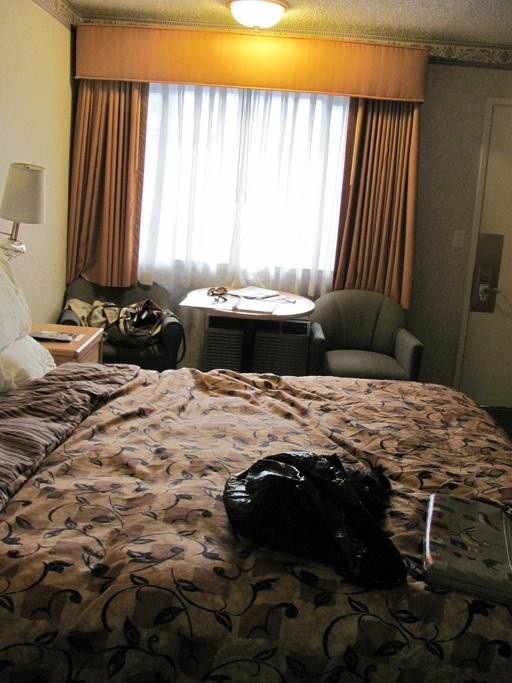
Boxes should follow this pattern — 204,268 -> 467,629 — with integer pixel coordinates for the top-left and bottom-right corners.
0,252 -> 57,396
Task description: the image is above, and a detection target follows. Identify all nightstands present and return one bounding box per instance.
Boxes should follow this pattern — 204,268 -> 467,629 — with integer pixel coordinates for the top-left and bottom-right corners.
30,321 -> 105,365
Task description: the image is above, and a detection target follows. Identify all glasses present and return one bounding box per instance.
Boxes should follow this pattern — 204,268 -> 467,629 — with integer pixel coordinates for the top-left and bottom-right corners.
207,287 -> 228,302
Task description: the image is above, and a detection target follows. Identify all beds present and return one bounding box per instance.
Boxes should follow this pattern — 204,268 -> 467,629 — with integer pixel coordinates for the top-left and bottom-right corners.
0,361 -> 512,682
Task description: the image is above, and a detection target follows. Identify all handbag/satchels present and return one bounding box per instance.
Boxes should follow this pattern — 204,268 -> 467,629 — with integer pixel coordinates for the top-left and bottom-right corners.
222,453 -> 408,589
85,298 -> 187,366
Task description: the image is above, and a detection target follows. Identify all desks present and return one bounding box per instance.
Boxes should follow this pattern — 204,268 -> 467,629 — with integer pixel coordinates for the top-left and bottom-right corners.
186,286 -> 316,374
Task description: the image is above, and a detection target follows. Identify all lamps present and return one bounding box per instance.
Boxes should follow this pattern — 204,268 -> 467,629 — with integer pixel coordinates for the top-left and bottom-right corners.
223,0 -> 290,33
0,161 -> 48,262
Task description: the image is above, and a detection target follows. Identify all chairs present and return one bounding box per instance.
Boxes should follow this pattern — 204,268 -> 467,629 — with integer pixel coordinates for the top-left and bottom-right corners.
308,289 -> 425,380
58,275 -> 184,371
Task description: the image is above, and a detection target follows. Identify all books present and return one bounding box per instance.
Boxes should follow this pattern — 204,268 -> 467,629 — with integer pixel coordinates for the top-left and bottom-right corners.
226,285 -> 279,299
179,292 -> 279,315
